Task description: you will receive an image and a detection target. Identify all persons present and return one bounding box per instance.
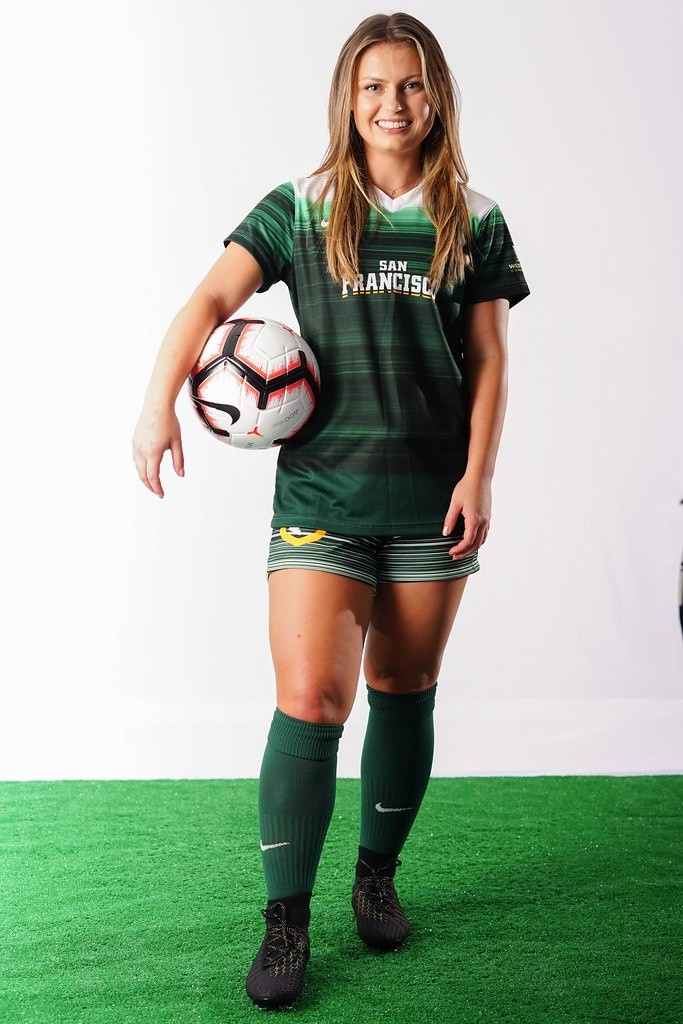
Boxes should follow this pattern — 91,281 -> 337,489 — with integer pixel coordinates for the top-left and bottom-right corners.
134,13 -> 531,1010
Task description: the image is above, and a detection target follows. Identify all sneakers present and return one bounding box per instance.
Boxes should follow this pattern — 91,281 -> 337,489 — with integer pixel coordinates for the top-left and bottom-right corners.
246,901 -> 311,1011
352,859 -> 410,948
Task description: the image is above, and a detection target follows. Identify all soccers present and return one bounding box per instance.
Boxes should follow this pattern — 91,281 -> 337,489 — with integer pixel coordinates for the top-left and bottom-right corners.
187,318 -> 321,451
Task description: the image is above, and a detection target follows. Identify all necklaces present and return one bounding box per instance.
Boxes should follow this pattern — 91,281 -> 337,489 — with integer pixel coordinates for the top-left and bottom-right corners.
372,178 -> 416,194
